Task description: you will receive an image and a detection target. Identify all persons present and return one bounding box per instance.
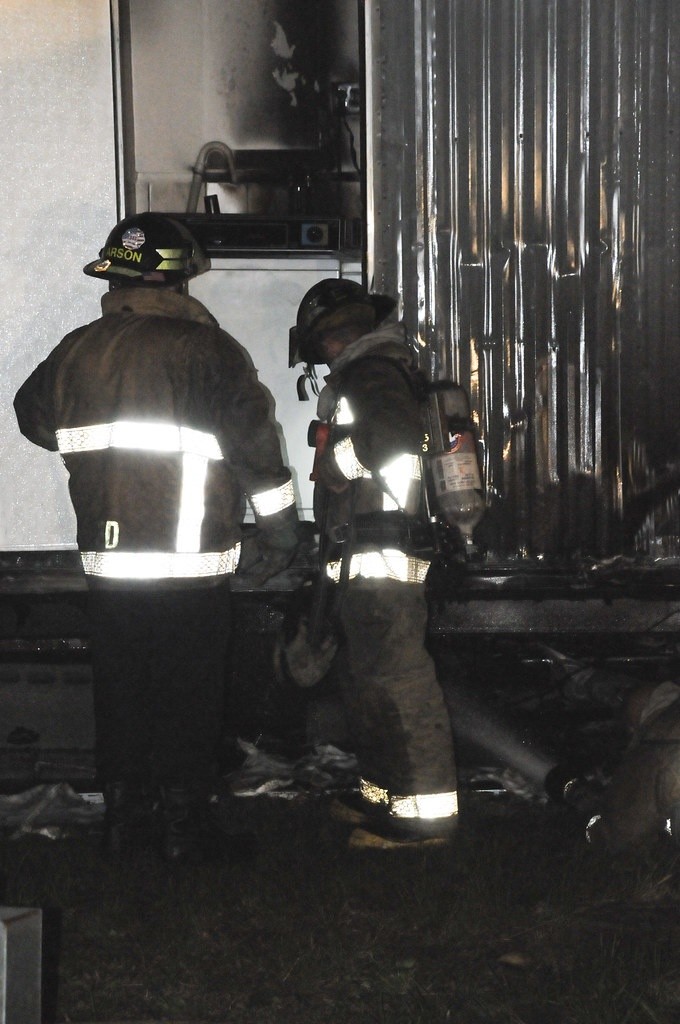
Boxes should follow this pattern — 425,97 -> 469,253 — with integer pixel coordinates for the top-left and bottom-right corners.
14,213 -> 300,873
300,278 -> 459,847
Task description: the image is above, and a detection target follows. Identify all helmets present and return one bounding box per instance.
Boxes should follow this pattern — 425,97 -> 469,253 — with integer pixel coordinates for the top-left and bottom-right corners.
294,278 -> 398,365
83,211 -> 212,288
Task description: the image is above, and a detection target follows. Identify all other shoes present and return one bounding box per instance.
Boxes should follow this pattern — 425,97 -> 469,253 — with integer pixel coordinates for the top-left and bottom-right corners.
325,788 -> 388,822
346,826 -> 455,851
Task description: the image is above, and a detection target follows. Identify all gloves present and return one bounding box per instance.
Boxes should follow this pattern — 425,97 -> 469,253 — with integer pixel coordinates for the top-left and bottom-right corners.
320,450 -> 354,495
234,502 -> 302,588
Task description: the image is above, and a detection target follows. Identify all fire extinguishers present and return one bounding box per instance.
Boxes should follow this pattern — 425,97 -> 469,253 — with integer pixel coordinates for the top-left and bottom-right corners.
422,378 -> 496,569
